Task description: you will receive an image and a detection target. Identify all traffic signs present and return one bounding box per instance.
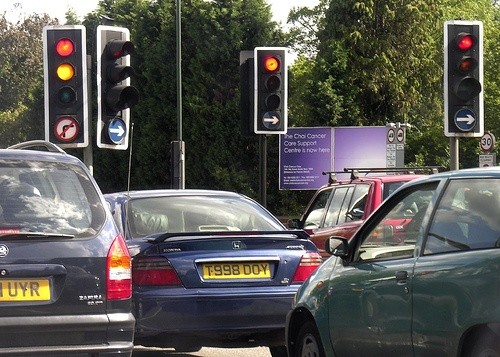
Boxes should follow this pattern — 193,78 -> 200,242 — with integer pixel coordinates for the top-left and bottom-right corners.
480,132 -> 493,151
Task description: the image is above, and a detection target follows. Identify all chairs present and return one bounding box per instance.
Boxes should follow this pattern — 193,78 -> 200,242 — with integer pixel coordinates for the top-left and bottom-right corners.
3,184 -> 42,222
423,207 -> 466,253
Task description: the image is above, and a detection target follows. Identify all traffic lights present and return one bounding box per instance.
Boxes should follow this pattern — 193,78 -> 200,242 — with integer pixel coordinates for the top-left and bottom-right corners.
96,25 -> 141,150
444,20 -> 483,136
42,25 -> 88,148
254,46 -> 288,134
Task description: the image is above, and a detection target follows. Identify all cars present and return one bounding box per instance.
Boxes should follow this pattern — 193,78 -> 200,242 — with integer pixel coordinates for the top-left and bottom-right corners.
103,189 -> 323,356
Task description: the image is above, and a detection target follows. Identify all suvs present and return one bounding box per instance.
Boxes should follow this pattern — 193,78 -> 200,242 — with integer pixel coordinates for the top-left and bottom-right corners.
287,166 -> 440,261
0,140 -> 136,357
285,165 -> 500,356
